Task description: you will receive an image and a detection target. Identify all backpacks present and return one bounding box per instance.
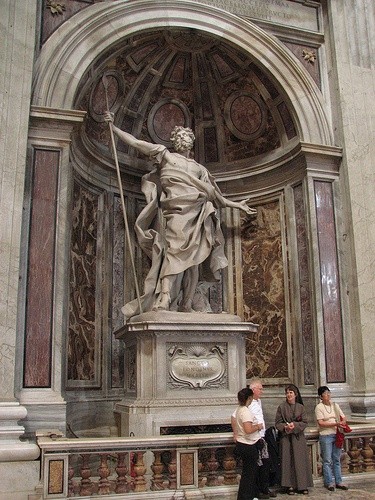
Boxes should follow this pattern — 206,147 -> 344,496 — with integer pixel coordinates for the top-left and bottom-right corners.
265,426 -> 280,467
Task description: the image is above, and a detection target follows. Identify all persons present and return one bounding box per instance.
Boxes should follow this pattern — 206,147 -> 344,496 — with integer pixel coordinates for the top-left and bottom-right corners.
275,384 -> 315,495
231,380 -> 277,499
101,110 -> 259,316
314,386 -> 348,492
235,388 -> 264,500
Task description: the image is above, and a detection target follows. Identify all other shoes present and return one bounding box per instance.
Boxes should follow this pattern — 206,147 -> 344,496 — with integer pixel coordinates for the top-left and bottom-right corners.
265,488 -> 276,497
296,489 -> 308,494
254,489 -> 269,499
324,485 -> 334,491
252,497 -> 258,500
287,487 -> 294,495
336,484 -> 348,490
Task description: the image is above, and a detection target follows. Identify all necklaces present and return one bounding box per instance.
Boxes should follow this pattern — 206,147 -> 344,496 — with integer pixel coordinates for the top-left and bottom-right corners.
322,401 -> 332,415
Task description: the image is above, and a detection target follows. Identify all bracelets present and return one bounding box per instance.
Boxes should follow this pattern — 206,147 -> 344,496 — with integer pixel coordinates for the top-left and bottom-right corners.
256,424 -> 259,434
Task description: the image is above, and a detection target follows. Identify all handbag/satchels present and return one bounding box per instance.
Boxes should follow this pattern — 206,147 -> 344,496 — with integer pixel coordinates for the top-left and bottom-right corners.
260,437 -> 269,460
335,426 -> 345,447
340,415 -> 352,432
